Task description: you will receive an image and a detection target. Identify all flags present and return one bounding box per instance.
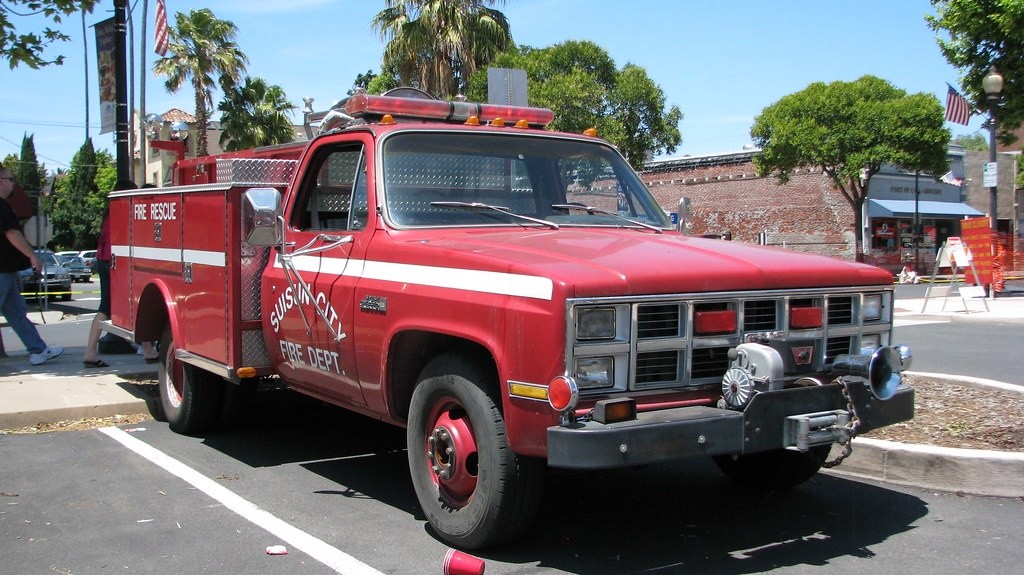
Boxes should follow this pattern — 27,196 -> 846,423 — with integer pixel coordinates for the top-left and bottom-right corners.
938,173 -> 963,186
91,17 -> 117,136
155,0 -> 168,57
945,85 -> 970,126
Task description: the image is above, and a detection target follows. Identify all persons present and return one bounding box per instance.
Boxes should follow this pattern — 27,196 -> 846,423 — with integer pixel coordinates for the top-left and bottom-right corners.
0,169 -> 65,365
82,179 -> 160,367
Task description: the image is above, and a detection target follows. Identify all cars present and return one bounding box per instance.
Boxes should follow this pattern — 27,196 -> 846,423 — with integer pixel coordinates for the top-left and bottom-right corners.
17,250 -> 98,301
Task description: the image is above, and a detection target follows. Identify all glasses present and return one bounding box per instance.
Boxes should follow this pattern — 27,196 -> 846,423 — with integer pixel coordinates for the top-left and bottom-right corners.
1,177 -> 14,181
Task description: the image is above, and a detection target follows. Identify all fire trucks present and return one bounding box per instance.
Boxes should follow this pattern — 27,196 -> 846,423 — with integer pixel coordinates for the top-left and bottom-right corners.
99,87 -> 918,556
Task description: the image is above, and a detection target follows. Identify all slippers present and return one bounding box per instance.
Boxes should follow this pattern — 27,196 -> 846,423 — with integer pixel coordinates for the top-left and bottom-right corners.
147,356 -> 159,363
84,359 -> 109,368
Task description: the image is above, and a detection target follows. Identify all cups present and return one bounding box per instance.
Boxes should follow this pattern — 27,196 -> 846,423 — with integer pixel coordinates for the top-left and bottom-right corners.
442,548 -> 485,575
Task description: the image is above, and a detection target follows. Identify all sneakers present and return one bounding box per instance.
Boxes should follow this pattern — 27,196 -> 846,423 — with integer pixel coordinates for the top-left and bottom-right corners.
30,346 -> 64,365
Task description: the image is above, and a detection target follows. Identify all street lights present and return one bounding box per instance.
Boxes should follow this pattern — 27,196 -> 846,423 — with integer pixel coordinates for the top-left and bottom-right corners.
982,59 -> 1002,269
